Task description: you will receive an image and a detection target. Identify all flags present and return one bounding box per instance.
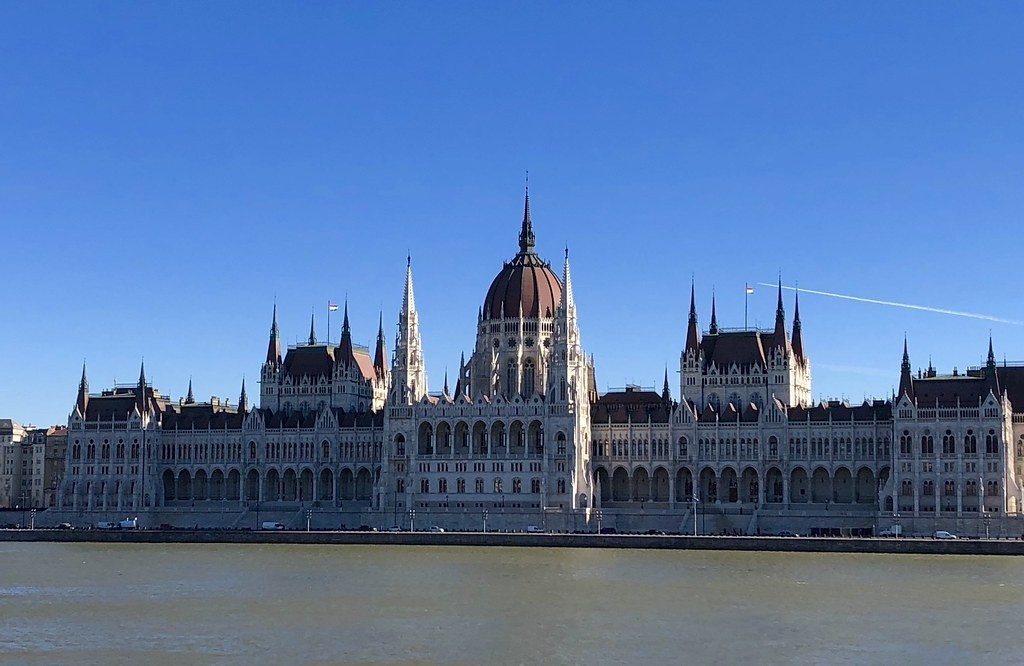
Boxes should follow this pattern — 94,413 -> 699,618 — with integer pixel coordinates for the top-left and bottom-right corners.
329,305 -> 338,311
747,287 -> 753,294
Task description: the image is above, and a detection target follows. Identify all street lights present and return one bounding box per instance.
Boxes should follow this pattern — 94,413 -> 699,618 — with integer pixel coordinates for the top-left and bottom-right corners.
30,509 -> 36,530
482,509 -> 489,533
409,509 -> 415,532
692,493 -> 699,536
595,511 -> 603,534
307,509 -> 313,531
983,514 -> 991,540
220,498 -> 228,519
893,513 -> 901,539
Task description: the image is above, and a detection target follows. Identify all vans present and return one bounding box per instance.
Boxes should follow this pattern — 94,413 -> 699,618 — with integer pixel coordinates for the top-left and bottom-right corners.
262,521 -> 285,531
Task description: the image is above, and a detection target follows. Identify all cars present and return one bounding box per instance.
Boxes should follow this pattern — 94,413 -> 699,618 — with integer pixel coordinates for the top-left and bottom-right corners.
387,525 -> 402,531
880,528 -> 902,537
527,526 -> 545,533
649,529 -> 665,535
935,531 -> 956,539
595,527 -> 616,534
425,526 -> 444,532
55,523 -> 71,529
159,523 -> 176,530
778,530 -> 796,537
358,525 -> 377,530
2,523 -> 21,529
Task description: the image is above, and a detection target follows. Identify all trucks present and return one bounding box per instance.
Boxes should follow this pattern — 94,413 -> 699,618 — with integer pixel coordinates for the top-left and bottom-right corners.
114,520 -> 136,530
96,522 -> 115,530
